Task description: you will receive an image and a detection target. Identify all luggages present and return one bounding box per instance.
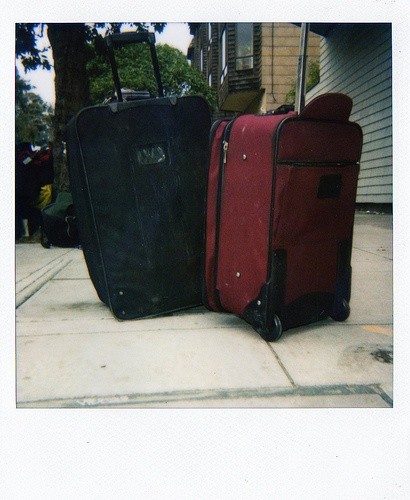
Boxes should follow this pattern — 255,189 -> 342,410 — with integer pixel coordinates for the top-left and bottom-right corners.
202,22 -> 363,341
66,31 -> 214,320
